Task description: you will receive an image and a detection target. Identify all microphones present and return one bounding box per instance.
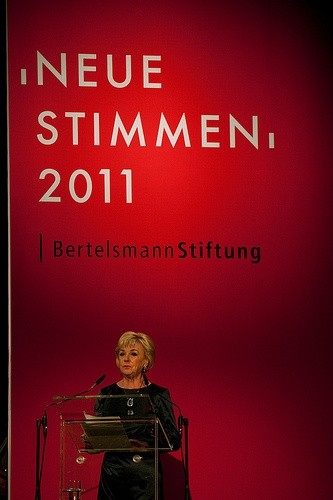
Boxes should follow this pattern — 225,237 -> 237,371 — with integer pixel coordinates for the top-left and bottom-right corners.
42,374 -> 107,438
143,374 -> 184,420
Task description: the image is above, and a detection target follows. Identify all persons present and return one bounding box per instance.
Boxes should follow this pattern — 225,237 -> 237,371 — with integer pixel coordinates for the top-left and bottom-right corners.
80,331 -> 182,500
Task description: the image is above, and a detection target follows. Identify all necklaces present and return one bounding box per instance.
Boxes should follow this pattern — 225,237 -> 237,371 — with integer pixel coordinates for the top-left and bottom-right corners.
122,378 -> 144,407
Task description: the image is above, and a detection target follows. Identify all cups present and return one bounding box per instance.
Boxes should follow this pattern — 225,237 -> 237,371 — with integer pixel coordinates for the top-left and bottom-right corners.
68,480 -> 81,500
